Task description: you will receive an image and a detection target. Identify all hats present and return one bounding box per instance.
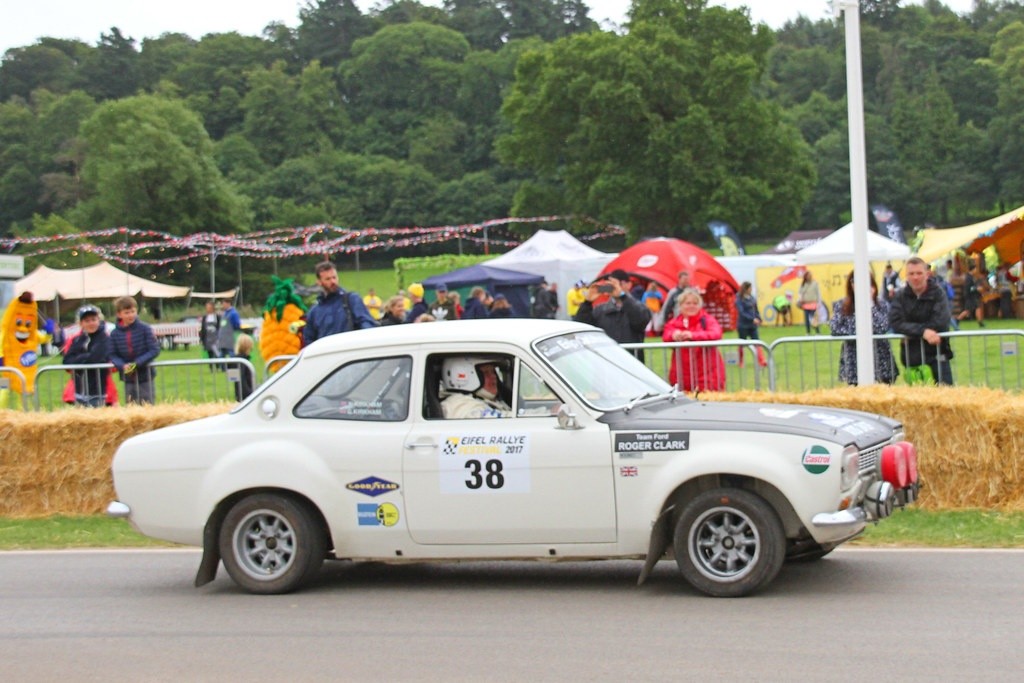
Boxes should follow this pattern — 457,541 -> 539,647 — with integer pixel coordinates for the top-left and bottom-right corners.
409,283 -> 424,297
436,282 -> 447,291
603,269 -> 629,282
79,306 -> 98,320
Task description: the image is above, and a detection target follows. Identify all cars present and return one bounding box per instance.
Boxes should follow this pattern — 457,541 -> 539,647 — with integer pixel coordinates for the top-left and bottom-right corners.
105,317 -> 925,594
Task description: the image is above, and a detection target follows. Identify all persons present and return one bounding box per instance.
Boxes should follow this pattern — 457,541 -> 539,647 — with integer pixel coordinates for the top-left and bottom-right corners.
437,355 -> 565,417
61,244 -> 1023,392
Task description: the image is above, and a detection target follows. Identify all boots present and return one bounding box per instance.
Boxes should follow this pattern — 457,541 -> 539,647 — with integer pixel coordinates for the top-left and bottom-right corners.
757,345 -> 768,366
738,347 -> 745,368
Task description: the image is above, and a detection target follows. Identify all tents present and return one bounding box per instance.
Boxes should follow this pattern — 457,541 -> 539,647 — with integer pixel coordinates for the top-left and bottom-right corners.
790,219 -> 911,265
418,226 -> 742,335
10,262 -> 240,336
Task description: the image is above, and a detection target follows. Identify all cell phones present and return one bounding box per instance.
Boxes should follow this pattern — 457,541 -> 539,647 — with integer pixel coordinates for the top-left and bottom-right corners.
598,285 -> 612,293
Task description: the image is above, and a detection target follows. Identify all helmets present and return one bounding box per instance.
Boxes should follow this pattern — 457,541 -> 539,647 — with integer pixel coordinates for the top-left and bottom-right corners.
441,352 -> 507,391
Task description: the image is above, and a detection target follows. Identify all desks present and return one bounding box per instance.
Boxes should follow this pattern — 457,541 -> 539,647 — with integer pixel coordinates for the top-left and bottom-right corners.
149,323 -> 202,349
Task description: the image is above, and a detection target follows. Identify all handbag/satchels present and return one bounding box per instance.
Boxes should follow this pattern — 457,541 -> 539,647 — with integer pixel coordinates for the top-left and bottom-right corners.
801,301 -> 819,311
797,300 -> 804,308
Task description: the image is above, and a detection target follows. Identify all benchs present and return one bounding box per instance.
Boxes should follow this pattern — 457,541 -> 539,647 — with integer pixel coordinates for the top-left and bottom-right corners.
174,336 -> 200,350
